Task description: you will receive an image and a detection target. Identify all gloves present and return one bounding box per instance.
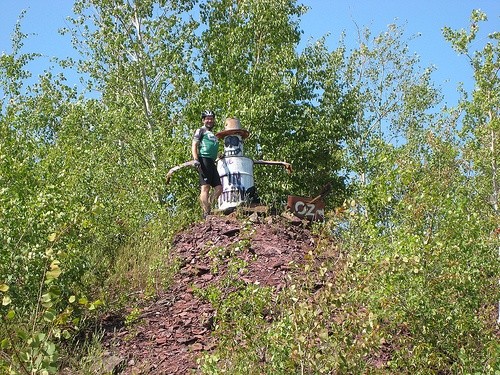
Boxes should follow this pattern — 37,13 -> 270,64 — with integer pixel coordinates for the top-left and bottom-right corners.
217,151 -> 226,158
194,159 -> 202,168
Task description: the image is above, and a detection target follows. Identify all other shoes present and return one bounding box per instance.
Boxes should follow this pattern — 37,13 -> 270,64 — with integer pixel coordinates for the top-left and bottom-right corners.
203,211 -> 212,219
210,193 -> 219,209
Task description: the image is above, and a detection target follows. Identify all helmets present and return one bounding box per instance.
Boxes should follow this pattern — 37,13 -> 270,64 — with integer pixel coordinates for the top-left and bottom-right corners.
202,109 -> 215,116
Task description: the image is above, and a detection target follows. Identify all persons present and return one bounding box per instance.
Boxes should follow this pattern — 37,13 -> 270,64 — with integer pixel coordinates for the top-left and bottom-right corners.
192,109 -> 224,216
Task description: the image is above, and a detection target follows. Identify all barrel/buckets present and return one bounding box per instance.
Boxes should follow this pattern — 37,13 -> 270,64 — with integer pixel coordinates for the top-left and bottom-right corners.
216,157 -> 254,214
